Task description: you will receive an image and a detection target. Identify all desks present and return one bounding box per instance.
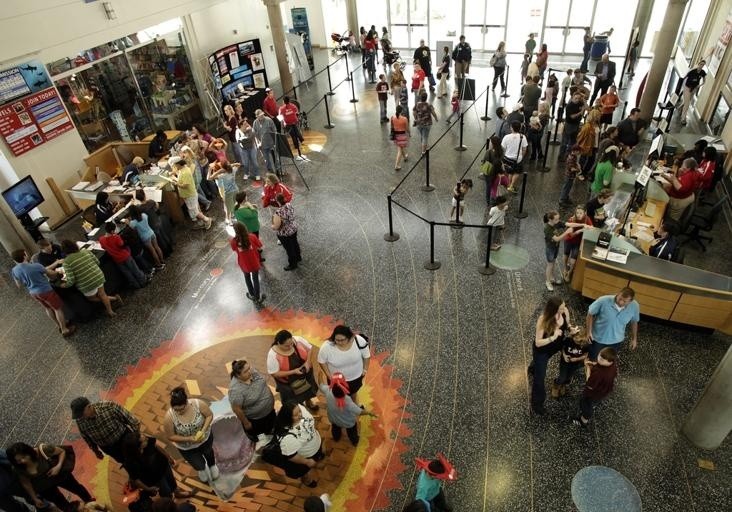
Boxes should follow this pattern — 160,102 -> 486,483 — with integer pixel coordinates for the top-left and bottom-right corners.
228,88 -> 268,125
630,157 -> 679,254
63,142 -> 185,222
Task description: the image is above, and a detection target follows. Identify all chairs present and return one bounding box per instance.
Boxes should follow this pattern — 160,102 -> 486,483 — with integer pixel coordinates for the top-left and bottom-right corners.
701,164 -> 724,197
83,204 -> 96,228
689,195 -> 727,252
670,244 -> 686,264
662,202 -> 695,239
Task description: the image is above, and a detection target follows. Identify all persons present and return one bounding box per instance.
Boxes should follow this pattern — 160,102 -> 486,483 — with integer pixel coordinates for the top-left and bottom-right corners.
360,25 -> 717,290
346,30 -> 356,54
11,86 -> 306,337
297,31 -> 314,71
403,451 -> 456,511
528,287 -> 639,426
0,324 -> 370,511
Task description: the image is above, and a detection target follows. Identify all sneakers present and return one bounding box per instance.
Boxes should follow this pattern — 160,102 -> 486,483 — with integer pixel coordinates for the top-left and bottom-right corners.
192,201 -> 234,230
199,403 -> 364,488
546,268 -> 570,291
255,176 -> 260,180
381,92 -> 451,170
508,185 -> 519,194
246,240 -> 297,300
244,175 -> 249,180
58,263 -> 166,337
534,385 -> 587,429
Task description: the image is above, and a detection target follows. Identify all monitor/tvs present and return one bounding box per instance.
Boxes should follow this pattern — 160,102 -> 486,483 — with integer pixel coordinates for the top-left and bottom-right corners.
1,175 -> 45,219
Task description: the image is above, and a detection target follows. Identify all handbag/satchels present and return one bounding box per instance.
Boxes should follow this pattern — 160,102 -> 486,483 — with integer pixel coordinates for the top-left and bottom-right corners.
504,157 -> 516,173
39,443 -> 75,472
292,378 -> 310,395
437,72 -> 449,79
480,161 -> 492,175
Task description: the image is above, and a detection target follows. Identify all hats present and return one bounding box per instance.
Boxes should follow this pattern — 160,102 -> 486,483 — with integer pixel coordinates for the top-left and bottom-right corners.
599,189 -> 612,197
